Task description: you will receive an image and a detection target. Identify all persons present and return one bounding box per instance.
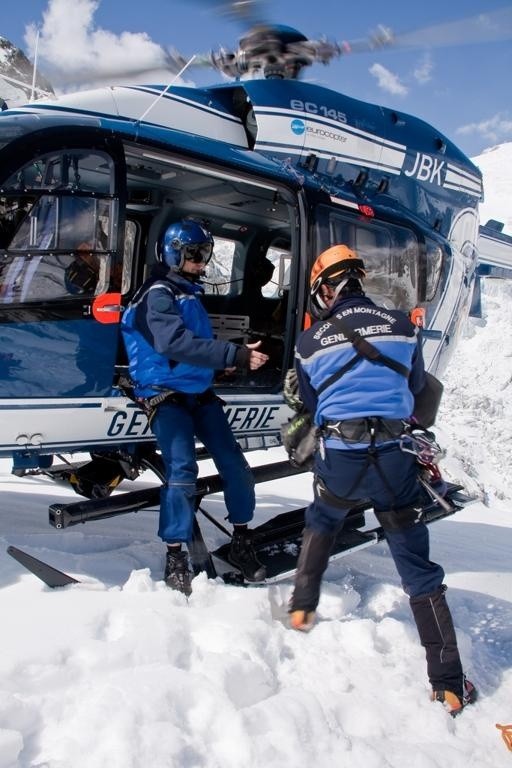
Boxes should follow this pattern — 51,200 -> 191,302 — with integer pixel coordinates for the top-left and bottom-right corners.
275,242 -> 478,719
121,219 -> 272,596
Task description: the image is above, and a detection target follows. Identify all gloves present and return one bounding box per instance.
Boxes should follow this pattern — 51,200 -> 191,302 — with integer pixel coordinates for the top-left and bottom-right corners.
233,344 -> 252,371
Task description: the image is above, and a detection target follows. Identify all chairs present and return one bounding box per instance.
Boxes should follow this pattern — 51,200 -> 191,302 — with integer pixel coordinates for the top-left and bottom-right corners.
231,256 -> 275,317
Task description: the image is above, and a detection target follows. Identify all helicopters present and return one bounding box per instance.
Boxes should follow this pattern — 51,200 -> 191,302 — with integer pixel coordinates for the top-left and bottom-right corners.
1,3 -> 511,583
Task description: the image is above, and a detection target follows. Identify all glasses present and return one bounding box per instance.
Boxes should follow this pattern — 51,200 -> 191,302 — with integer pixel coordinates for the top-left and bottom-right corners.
183,247 -> 212,265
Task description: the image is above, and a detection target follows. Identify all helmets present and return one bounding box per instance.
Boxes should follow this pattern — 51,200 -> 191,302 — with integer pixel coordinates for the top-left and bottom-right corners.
162,220 -> 214,272
309,244 -> 367,313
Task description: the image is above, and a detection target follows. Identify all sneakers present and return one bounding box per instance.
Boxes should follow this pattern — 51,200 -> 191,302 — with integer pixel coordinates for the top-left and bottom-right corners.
432,679 -> 478,718
288,596 -> 316,630
164,550 -> 193,595
227,533 -> 269,582
